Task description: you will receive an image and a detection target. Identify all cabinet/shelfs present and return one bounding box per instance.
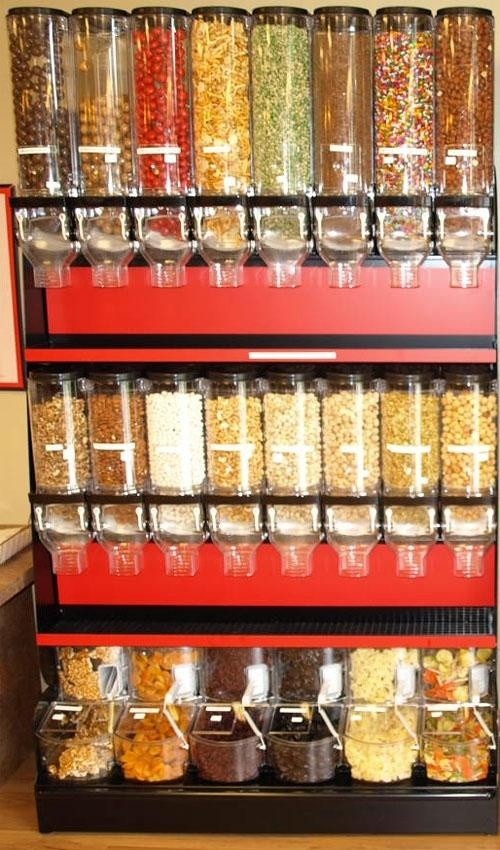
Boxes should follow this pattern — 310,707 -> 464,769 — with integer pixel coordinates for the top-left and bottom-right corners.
10,193 -> 500,836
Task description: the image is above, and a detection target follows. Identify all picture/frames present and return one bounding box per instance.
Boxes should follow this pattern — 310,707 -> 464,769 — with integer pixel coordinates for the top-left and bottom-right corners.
0,182 -> 27,392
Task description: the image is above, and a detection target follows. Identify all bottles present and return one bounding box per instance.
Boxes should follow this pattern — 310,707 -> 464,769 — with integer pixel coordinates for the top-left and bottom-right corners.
6,8 -> 496,290
51,647 -> 498,703
25,367 -> 497,577
